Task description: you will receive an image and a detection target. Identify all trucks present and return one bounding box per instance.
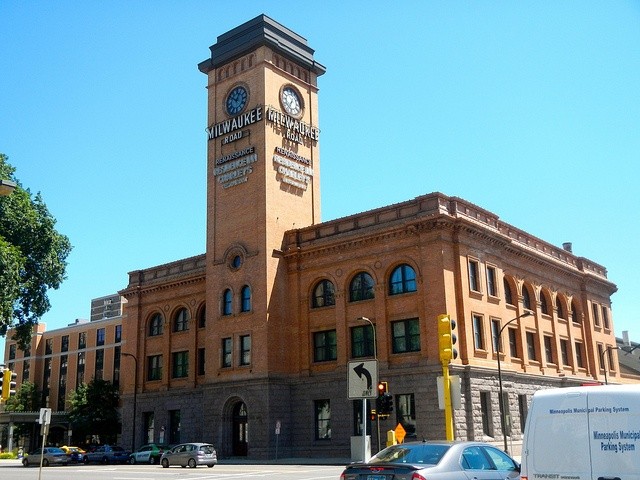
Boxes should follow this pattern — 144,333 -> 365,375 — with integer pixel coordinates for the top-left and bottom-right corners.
520,385 -> 640,480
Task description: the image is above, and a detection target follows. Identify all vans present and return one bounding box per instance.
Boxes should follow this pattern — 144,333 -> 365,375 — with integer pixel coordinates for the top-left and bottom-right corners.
160,442 -> 217,468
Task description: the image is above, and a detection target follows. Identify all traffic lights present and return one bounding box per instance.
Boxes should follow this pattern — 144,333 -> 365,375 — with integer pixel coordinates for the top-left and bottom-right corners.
376,395 -> 394,415
2,369 -> 17,400
0,372 -> 3,394
438,314 -> 458,361
371,414 -> 378,422
377,381 -> 388,395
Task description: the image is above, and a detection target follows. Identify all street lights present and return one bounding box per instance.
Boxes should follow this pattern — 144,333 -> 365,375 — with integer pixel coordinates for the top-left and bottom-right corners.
497,312 -> 533,456
601,345 -> 633,385
122,353 -> 138,453
357,316 -> 381,452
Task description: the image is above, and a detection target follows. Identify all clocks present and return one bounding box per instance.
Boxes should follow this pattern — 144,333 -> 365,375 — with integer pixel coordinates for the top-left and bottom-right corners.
221,80 -> 250,119
279,82 -> 306,123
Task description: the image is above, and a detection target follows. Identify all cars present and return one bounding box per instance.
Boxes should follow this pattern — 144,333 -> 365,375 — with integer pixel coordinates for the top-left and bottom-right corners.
59,446 -> 86,464
22,447 -> 68,466
83,444 -> 131,464
129,443 -> 170,465
339,435 -> 521,480
